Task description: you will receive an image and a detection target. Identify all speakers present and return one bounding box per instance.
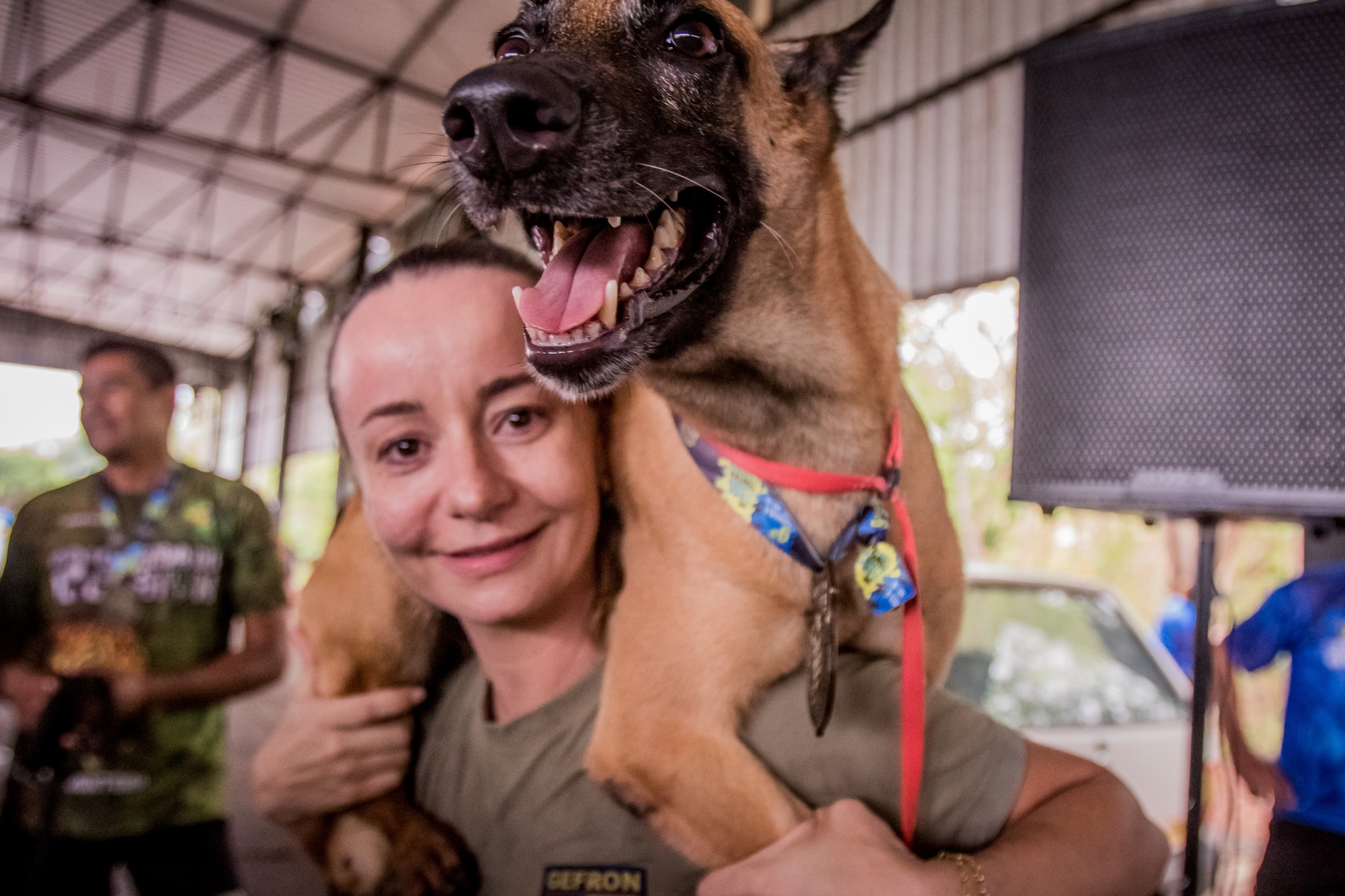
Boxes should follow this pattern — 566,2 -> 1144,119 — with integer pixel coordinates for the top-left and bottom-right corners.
1008,0 -> 1345,520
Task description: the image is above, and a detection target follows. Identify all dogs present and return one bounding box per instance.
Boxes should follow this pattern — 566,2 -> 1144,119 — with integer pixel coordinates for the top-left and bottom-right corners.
276,1 -> 968,896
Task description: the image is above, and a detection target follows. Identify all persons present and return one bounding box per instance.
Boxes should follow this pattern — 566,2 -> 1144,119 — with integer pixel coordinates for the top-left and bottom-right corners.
0,335 -> 289,896
1160,582 -> 1222,841
1207,569 -> 1345,896
245,238 -> 1173,896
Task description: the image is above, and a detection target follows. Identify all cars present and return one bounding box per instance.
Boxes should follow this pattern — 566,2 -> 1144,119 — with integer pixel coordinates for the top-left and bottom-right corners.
943,560 -> 1241,896
